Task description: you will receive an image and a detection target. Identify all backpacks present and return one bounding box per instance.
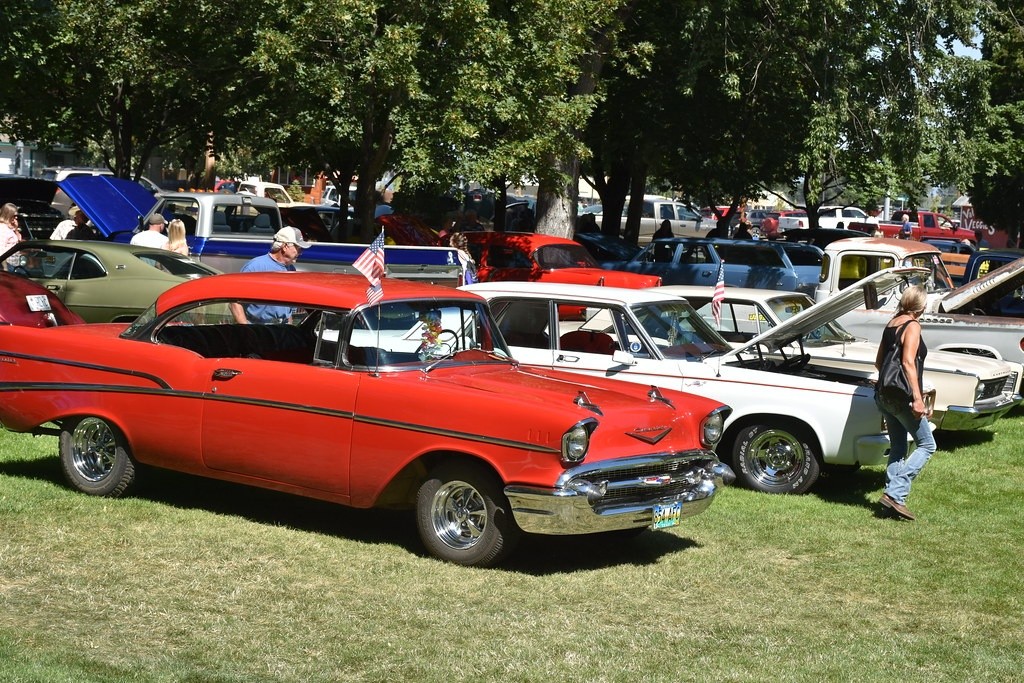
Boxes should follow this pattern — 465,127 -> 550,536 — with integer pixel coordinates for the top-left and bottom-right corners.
458,252 -> 478,285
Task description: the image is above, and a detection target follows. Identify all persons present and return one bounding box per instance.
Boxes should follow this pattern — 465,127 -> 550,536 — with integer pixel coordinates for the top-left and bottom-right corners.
228,226 -> 312,324
447,232 -> 478,284
1006,231 -> 1018,249
706,221 -> 727,239
66,211 -> 96,240
734,222 -> 752,240
440,200 -> 485,266
0,203 -> 21,271
975,230 -> 989,251
130,213 -> 169,265
898,214 -> 912,242
506,206 -> 600,233
155,221 -> 188,273
871,285 -> 937,521
651,220 -> 674,263
49,207 -> 80,240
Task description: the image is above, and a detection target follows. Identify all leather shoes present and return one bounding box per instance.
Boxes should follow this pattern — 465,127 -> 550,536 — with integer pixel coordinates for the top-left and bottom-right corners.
879,493 -> 915,520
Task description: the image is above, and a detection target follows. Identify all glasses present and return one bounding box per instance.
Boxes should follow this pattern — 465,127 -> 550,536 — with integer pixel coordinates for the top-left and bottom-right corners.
11,214 -> 20,219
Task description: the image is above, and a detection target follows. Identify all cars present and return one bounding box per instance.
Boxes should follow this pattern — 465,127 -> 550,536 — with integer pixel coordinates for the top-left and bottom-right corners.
0,140 -> 1024,409
544,283 -> 1022,438
312,268 -> 949,499
0,271 -> 738,569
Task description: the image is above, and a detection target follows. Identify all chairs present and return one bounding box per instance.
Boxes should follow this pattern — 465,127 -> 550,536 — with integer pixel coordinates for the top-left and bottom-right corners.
558,330 -> 614,354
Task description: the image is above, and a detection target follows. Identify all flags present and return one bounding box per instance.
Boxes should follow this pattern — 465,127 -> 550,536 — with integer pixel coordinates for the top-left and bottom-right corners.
352,230 -> 385,306
711,262 -> 725,326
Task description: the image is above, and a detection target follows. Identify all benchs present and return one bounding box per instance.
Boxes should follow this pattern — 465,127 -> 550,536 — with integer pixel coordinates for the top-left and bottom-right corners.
159,322 -> 321,364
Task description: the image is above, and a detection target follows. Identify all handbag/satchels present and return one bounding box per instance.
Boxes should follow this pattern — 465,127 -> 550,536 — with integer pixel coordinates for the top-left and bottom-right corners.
879,320 -> 914,400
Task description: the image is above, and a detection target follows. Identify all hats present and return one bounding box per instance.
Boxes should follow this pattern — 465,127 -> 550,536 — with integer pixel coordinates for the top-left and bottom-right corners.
276,226 -> 313,249
149,213 -> 168,225
